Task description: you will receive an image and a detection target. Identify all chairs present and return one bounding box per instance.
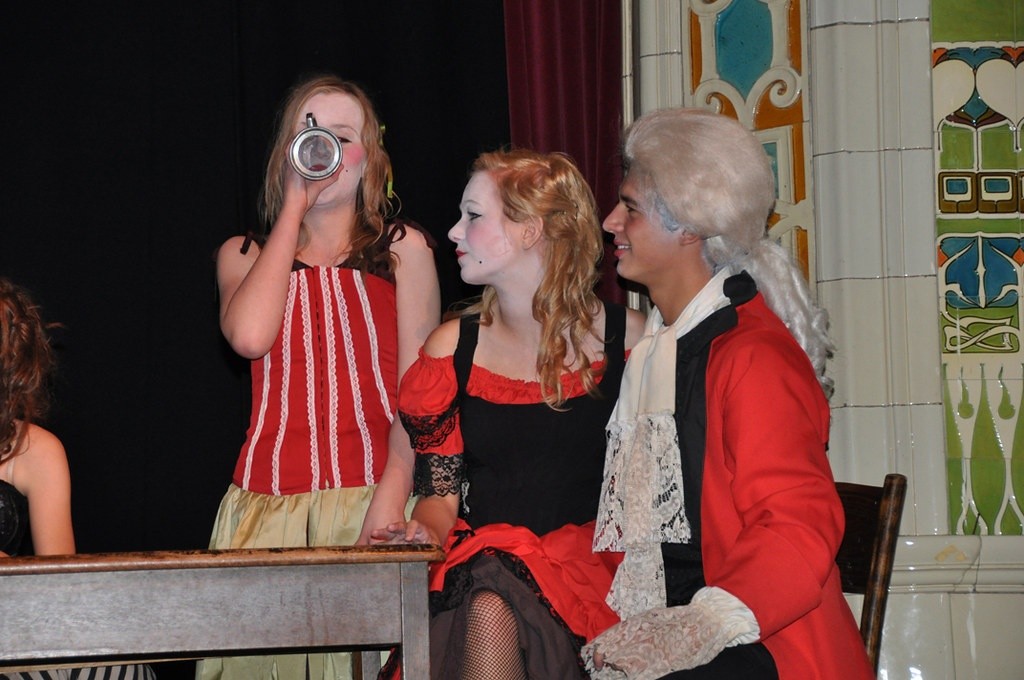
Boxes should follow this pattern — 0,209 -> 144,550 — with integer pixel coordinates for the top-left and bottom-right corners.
835,474 -> 909,675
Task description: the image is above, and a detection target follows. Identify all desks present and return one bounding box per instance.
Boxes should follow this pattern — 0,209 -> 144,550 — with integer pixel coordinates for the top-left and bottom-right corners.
1,542 -> 448,680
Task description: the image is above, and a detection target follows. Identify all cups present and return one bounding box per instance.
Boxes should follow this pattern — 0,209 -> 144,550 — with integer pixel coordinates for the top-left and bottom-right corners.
289,113 -> 343,181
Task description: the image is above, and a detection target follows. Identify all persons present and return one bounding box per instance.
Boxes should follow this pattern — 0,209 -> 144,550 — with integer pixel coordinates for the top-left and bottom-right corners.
0,276 -> 161,680
198,74 -> 441,680
369,145 -> 649,680
578,105 -> 877,680
